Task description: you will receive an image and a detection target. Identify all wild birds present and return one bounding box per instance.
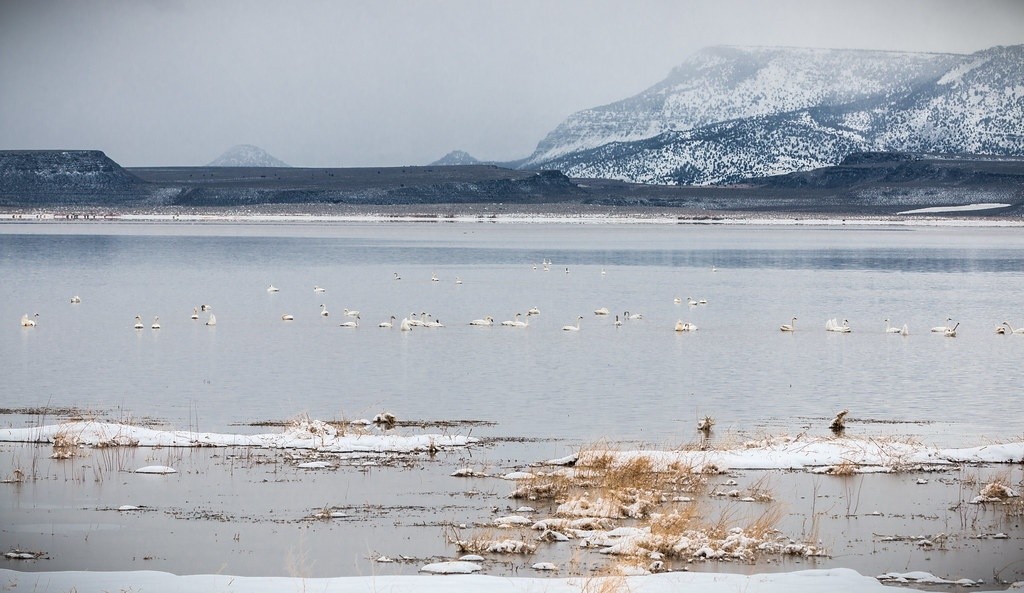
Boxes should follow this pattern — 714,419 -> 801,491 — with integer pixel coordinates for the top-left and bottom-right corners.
778,315 -> 1024,338
21,312 -> 40,327
133,305 -> 218,329
69,296 -> 81,304
266,259 -> 719,332
